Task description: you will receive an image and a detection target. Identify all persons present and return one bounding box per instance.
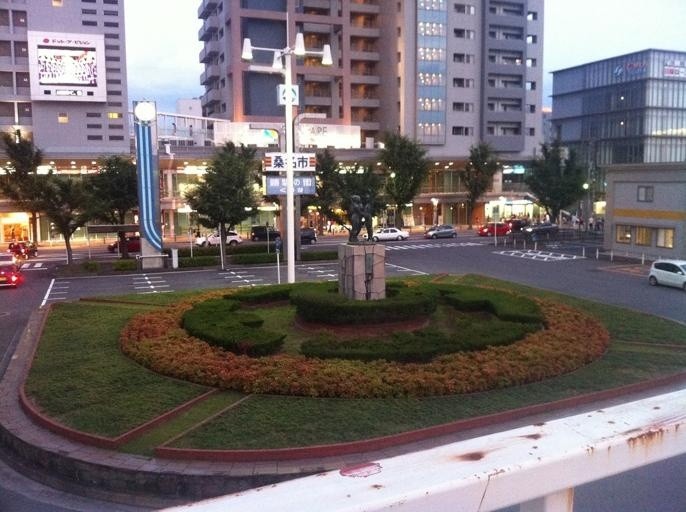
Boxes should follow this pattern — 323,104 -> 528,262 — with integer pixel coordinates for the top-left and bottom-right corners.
172,123 -> 176,134
11,228 -> 16,242
485,211 -> 605,232
189,124 -> 193,136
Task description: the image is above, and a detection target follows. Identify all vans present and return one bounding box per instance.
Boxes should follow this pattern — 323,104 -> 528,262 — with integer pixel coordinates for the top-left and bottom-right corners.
8,241 -> 39,259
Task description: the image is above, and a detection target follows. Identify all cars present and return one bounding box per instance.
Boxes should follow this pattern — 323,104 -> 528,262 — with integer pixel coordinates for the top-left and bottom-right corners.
0,254 -> 23,271
478,221 -> 512,237
648,259 -> 686,290
362,228 -> 410,241
520,222 -> 560,235
423,225 -> 458,238
507,219 -> 525,232
0,265 -> 22,288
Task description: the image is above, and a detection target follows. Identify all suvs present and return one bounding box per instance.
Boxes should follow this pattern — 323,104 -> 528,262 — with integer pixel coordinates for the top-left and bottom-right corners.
275,228 -> 318,247
250,224 -> 281,241
195,229 -> 243,248
106,235 -> 139,253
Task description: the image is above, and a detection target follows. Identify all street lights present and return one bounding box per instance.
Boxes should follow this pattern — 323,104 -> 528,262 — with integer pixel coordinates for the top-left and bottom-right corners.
237,34 -> 333,284
583,167 -> 591,248
